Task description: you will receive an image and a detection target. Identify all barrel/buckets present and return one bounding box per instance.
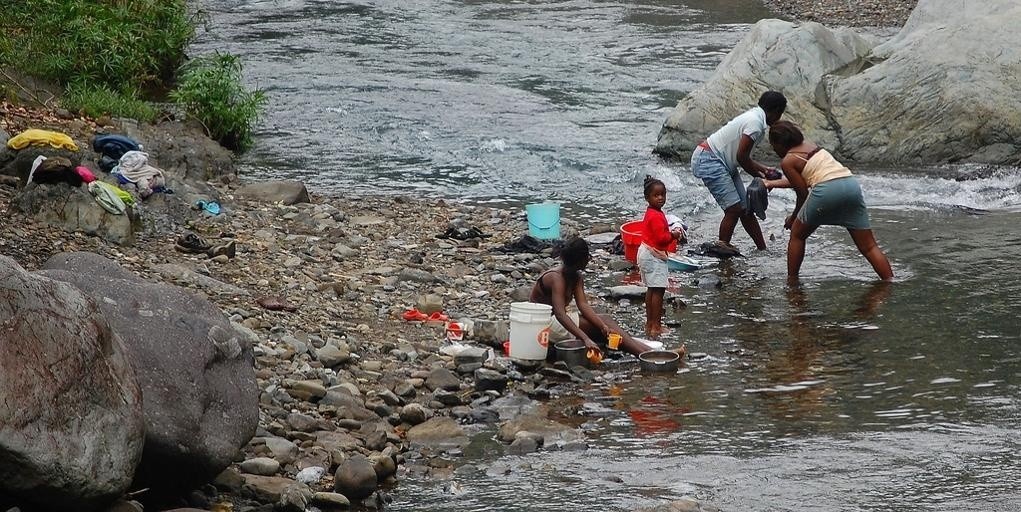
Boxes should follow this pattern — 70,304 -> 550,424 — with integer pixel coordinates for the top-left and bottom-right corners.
509,302 -> 552,362
525,202 -> 560,240
619,221 -> 643,260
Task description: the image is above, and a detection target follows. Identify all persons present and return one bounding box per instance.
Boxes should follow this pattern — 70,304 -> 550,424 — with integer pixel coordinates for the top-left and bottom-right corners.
690,91 -> 788,251
749,121 -> 895,279
637,175 -> 681,336
530,237 -> 684,360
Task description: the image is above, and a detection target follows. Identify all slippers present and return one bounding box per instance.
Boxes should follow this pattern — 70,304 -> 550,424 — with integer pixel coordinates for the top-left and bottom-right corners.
429,311 -> 449,321
402,308 -> 429,321
460,227 -> 493,239
205,200 -> 221,215
435,226 -> 464,241
191,199 -> 208,210
446,323 -> 469,332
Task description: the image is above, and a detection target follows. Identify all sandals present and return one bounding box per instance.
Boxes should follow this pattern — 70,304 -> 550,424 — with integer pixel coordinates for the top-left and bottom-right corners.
175,231 -> 211,254
208,241 -> 235,257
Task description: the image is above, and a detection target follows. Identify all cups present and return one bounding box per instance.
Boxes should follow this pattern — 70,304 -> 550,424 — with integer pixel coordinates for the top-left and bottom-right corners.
608,334 -> 622,349
586,349 -> 602,365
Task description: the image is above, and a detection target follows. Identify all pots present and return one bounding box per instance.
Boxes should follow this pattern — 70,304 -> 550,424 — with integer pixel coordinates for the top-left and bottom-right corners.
638,349 -> 680,372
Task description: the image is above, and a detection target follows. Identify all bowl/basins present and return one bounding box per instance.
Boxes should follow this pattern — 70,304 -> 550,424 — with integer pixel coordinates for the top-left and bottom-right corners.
667,252 -> 704,273
